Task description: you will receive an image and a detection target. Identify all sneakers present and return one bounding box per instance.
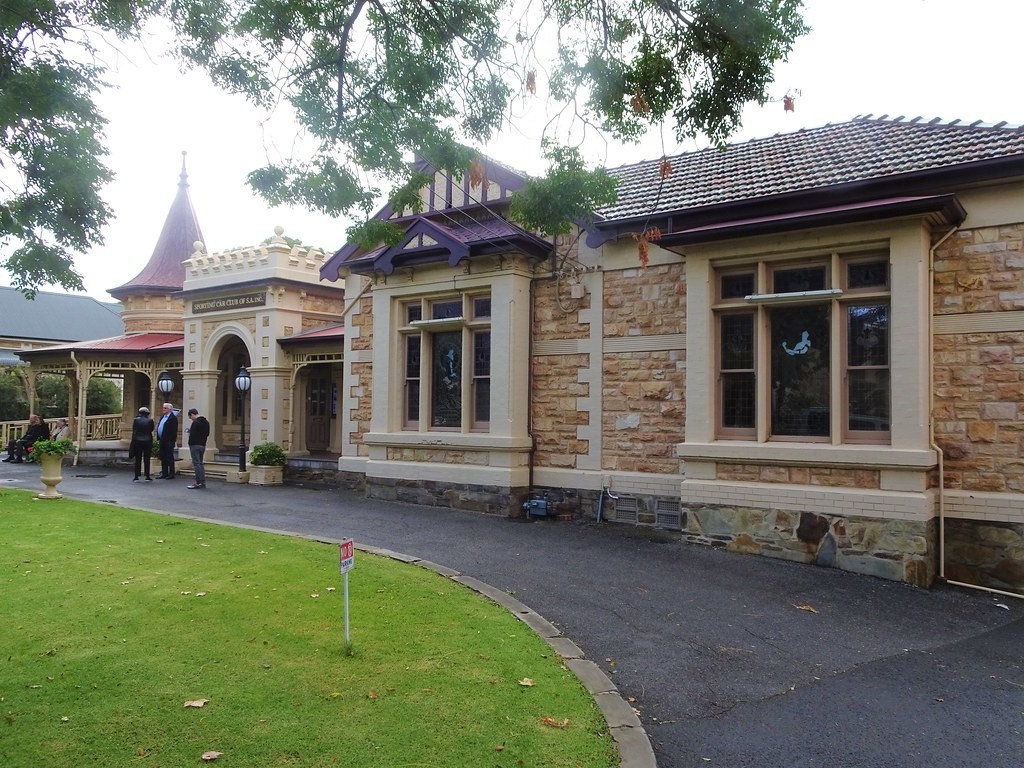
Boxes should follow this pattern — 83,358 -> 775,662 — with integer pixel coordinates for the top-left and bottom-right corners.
187,483 -> 206,489
144,478 -> 151,482
133,478 -> 137,482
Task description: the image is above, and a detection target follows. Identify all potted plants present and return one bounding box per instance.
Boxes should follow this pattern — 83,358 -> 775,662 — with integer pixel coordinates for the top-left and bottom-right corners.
248,441 -> 288,485
141,438 -> 170,475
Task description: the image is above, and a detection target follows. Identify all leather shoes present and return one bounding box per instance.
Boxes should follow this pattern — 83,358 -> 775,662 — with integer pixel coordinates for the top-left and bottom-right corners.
165,475 -> 174,479
1,456 -> 15,462
155,474 -> 165,478
10,456 -> 22,463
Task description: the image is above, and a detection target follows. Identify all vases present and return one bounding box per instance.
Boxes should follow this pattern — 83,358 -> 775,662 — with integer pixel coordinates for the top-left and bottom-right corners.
27,453 -> 78,499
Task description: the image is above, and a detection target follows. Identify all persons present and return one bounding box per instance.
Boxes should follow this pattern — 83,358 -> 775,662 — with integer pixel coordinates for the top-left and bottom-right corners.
52,418 -> 70,442
2,414 -> 38,463
156,403 -> 178,479
187,409 -> 210,488
133,407 -> 154,483
25,416 -> 49,463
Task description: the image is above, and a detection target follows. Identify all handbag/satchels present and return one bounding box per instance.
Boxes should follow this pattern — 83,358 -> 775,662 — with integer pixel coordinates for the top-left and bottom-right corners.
127,440 -> 134,458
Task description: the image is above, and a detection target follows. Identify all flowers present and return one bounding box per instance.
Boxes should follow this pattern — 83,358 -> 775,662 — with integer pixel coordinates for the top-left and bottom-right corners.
27,434 -> 78,453
